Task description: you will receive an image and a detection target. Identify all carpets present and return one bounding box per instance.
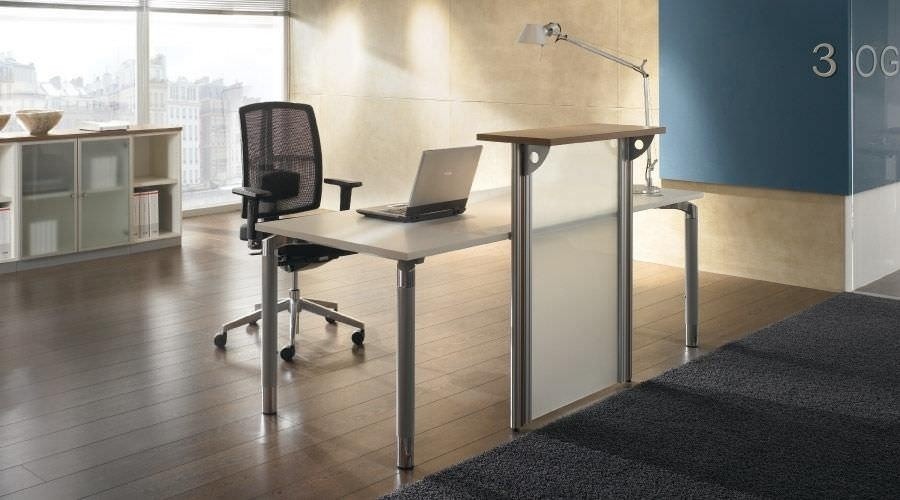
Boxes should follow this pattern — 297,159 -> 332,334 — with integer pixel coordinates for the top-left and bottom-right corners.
379,291 -> 900,498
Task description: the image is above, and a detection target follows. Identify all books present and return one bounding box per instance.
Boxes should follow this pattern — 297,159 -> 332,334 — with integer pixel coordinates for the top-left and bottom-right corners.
131,190 -> 161,244
88,155 -> 119,191
80,122 -> 130,131
29,213 -> 65,256
1,207 -> 13,260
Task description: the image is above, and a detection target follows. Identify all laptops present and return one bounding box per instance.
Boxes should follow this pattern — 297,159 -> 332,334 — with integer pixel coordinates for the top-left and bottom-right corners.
355,144 -> 483,224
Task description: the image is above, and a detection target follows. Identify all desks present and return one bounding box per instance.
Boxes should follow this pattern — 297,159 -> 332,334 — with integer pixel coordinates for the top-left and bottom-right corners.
253,184 -> 703,469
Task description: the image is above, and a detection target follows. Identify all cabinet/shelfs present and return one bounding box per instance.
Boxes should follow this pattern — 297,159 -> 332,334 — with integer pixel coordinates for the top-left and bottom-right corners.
0,139 -> 21,274
22,128 -> 131,272
131,125 -> 181,255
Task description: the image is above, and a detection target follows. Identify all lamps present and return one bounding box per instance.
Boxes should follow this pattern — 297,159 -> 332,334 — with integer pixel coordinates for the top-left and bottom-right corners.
516,21 -> 661,197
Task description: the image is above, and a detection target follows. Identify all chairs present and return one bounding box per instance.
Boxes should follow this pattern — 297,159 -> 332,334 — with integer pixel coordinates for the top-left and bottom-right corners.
213,102 -> 368,360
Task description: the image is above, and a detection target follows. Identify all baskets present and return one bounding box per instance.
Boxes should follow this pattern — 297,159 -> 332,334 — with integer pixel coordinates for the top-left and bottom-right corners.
0,114 -> 11,131
